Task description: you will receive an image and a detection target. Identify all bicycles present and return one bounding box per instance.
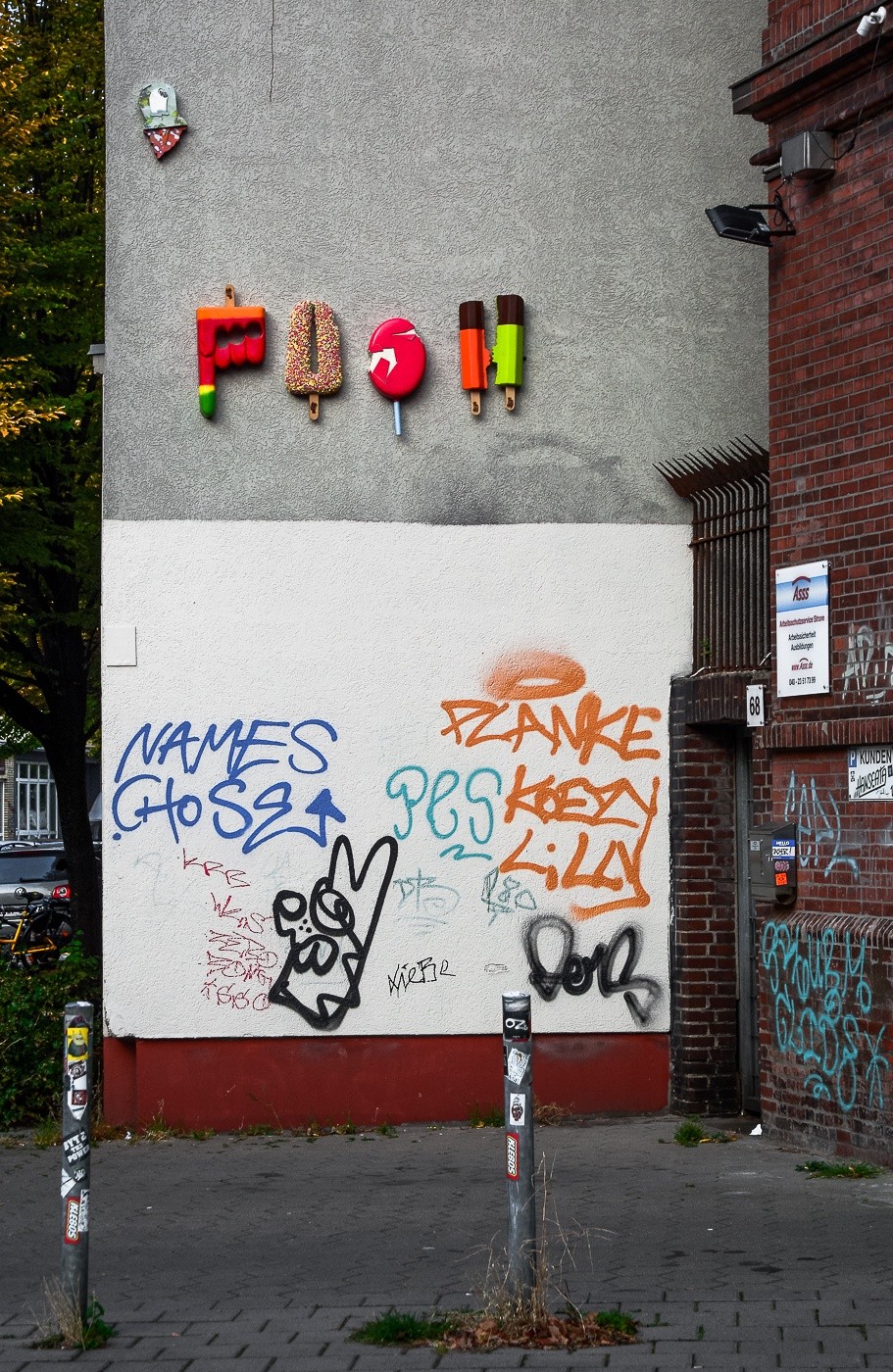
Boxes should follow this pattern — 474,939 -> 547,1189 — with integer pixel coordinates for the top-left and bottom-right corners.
0,891 -> 76,975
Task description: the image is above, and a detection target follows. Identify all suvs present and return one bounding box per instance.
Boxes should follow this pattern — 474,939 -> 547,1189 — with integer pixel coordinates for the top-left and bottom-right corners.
0,839 -> 103,950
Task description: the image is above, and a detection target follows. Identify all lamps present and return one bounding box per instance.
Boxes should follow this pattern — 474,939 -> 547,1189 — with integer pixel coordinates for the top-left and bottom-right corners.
705,194 -> 796,250
